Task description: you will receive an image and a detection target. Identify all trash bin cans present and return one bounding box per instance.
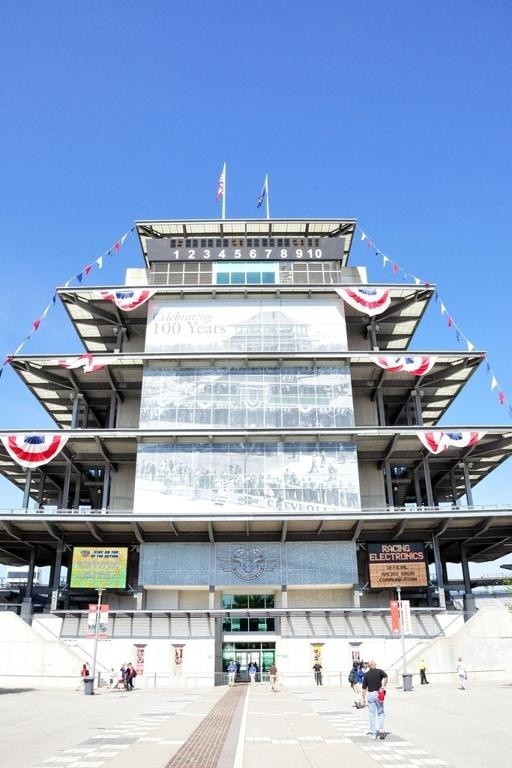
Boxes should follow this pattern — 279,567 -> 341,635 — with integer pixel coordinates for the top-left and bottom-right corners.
403,675 -> 412,691
84,678 -> 94,695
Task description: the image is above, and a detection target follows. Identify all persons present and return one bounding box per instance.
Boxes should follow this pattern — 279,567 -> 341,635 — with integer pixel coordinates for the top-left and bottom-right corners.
312,661 -> 323,686
269,662 -> 278,692
418,657 -> 429,685
227,660 -> 240,687
109,661 -> 139,691
457,655 -> 468,690
74,663 -> 89,692
249,663 -> 256,687
346,660 -> 389,741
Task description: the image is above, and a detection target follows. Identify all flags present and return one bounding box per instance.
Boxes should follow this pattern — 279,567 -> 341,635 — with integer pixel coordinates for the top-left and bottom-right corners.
256,174 -> 270,207
214,159 -> 226,200
